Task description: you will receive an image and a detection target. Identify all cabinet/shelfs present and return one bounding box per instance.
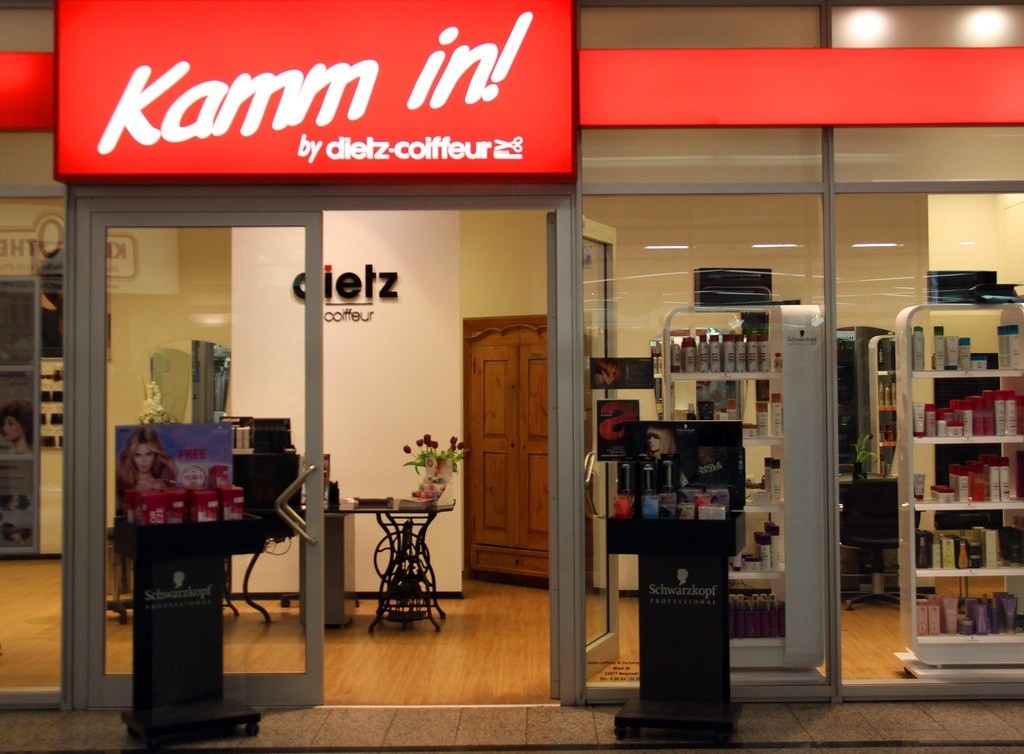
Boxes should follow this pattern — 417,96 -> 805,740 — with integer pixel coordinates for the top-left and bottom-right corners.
663,364 -> 787,668
879,369 -> 898,448
914,351 -> 1024,666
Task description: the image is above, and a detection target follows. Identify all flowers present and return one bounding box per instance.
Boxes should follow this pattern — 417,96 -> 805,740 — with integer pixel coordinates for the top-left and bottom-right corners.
402,433 -> 473,475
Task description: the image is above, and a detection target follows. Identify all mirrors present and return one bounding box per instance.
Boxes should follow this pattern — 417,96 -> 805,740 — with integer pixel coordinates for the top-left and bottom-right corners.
837,325 -> 896,479
139,338 -> 237,424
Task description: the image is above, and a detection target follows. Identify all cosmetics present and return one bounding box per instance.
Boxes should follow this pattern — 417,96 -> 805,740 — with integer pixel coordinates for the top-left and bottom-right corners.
880,323 -> 1024,636
613,331 -> 785,640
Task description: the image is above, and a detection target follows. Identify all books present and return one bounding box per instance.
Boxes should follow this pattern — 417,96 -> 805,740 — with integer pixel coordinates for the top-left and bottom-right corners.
233,425 -> 251,448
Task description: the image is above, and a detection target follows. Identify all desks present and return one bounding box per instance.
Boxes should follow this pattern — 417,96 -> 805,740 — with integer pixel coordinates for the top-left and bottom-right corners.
220,496 -> 456,634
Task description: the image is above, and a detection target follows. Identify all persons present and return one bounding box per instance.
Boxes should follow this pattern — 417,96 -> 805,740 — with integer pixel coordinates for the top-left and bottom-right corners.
0,494 -> 31,543
0,398 -> 34,455
647,425 -> 675,459
116,427 -> 179,489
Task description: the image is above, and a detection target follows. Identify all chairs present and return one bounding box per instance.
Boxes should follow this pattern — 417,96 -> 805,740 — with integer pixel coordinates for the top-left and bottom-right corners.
838,479 -> 923,613
